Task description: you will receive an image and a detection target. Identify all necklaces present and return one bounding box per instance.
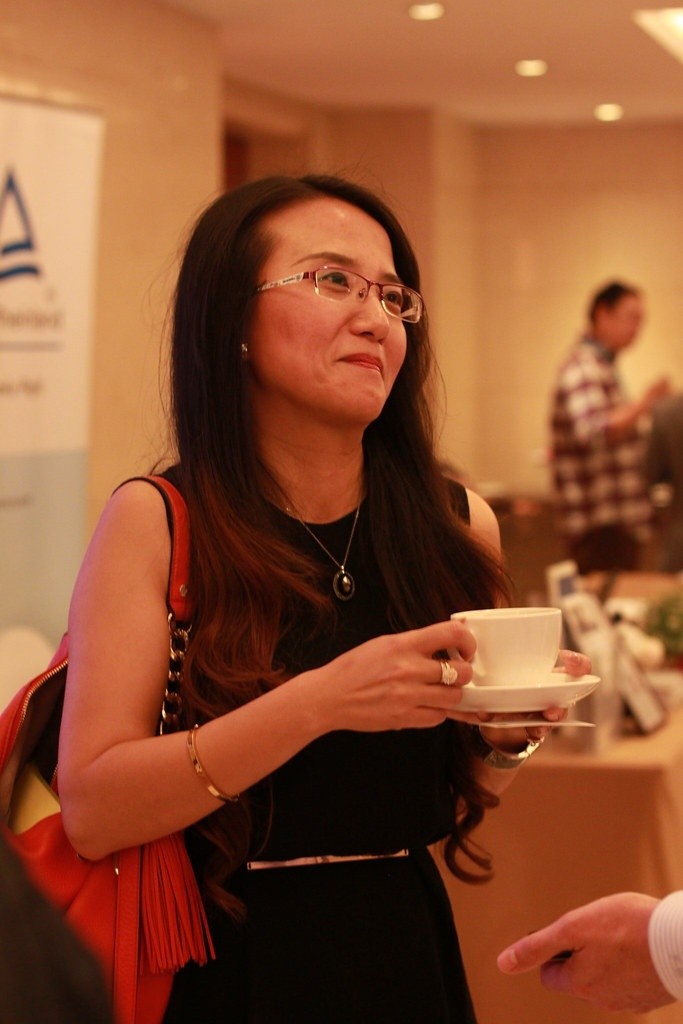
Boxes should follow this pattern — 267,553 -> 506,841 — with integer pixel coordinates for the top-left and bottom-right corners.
278,499 -> 364,602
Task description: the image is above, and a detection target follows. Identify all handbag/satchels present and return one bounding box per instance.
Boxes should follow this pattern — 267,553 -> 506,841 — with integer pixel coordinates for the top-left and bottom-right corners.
0,475 -> 215,1024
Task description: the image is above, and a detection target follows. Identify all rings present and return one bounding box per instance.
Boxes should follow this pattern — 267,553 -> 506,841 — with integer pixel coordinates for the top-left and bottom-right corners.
439,660 -> 459,687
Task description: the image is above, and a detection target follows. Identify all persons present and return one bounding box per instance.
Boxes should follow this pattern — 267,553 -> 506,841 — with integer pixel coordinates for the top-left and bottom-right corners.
56,172 -> 592,1023
497,888 -> 683,1018
548,278 -> 670,576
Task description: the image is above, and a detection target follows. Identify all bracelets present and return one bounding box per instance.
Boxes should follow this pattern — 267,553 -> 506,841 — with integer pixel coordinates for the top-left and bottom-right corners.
184,722 -> 241,803
472,731 -> 545,769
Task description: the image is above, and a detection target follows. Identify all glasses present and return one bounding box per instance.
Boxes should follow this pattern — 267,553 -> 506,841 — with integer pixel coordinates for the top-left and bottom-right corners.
255,266 -> 424,324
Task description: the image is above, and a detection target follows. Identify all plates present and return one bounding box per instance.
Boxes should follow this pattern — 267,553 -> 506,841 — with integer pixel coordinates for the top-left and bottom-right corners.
457,672 -> 602,712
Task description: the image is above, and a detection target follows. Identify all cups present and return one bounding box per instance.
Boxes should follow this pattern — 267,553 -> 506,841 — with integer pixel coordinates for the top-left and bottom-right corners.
449,608 -> 561,687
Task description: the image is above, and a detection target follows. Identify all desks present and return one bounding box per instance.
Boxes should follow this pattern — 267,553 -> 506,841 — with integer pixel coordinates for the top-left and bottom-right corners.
427,669 -> 683,1024
